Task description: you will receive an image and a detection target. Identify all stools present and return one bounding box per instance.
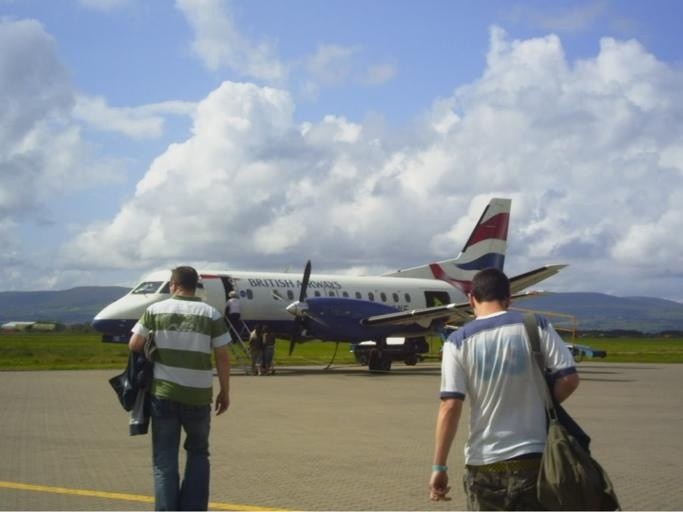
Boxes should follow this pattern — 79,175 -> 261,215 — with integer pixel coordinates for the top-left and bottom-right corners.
478,460 -> 538,473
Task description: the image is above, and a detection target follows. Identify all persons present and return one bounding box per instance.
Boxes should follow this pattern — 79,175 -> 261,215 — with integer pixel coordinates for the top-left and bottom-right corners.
429,267 -> 580,511
261,325 -> 275,376
226,290 -> 240,343
128,267 -> 230,511
249,325 -> 265,376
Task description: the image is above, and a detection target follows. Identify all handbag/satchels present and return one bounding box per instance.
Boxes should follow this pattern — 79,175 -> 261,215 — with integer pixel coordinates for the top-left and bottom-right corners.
431,465 -> 447,472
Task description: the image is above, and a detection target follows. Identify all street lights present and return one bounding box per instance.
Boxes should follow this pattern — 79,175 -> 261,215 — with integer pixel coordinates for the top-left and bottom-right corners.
537,417 -> 622,510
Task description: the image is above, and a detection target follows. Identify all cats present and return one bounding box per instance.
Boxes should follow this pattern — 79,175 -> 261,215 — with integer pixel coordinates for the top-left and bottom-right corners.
91,197 -> 570,374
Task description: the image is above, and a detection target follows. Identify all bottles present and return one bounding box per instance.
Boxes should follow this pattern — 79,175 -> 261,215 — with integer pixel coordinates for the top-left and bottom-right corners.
228,291 -> 237,297
